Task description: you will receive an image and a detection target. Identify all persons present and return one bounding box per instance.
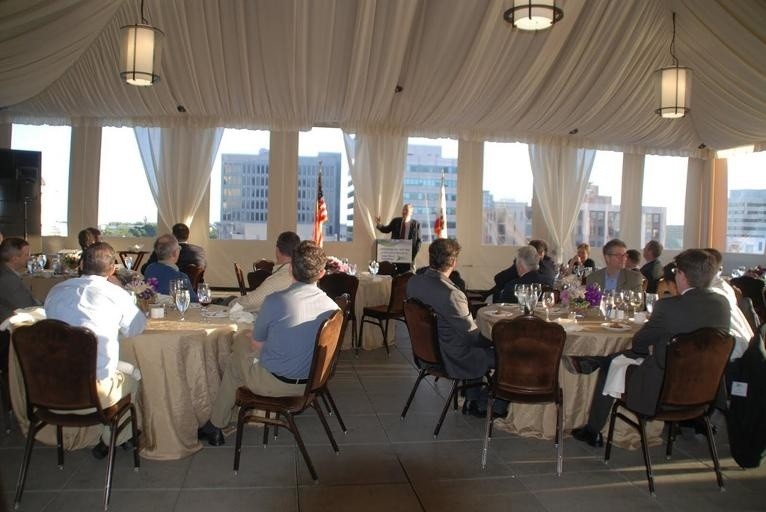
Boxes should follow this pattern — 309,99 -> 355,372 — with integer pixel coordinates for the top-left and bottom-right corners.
0,238 -> 43,329
562,249 -> 730,447
642,242 -> 665,295
78,228 -> 107,275
587,240 -> 643,309
144,234 -> 199,304
141,223 -> 206,289
568,243 -> 595,275
376,204 -> 422,273
45,242 -> 146,459
197,241 -> 341,448
407,239 -> 506,419
625,251 -> 642,274
229,232 -> 301,323
415,240 -> 465,294
704,249 -> 736,336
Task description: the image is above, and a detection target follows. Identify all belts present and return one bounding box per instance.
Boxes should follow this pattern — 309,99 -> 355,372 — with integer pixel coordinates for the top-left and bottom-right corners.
271,372 -> 308,385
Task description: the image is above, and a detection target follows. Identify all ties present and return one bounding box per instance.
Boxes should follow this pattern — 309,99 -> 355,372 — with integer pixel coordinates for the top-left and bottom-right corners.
399,219 -> 405,239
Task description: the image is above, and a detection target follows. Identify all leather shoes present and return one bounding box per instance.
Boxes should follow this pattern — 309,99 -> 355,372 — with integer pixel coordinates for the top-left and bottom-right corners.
93,434 -> 108,460
564,355 -> 582,376
198,427 -> 225,446
462,399 -> 508,419
122,430 -> 142,451
572,425 -> 603,448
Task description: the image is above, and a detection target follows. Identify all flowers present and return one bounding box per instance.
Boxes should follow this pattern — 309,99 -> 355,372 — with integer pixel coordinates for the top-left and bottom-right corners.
325,255 -> 349,274
62,253 -> 81,270
559,280 -> 603,307
124,274 -> 158,299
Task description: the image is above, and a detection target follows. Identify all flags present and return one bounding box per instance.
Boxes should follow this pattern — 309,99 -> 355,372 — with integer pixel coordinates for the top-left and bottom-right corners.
314,167 -> 328,246
438,181 -> 447,238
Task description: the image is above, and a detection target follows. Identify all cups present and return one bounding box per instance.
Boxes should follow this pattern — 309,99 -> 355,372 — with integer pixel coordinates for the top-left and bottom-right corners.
541,291 -> 556,323
732,269 -> 740,278
634,311 -> 646,321
346,262 -> 358,276
369,263 -> 380,277
169,280 -> 181,311
197,283 -> 209,314
199,289 -> 211,316
125,256 -> 133,271
176,289 -> 190,322
368,260 -> 376,277
151,307 -> 165,321
27,261 -> 36,274
601,289 -> 642,321
39,254 -> 47,270
645,293 -> 659,313
512,281 -> 542,318
738,266 -> 745,277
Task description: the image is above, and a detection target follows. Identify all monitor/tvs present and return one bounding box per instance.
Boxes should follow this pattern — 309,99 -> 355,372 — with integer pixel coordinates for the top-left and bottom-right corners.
1,147 -> 41,238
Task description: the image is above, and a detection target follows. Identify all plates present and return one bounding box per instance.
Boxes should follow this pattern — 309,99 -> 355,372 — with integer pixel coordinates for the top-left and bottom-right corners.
566,324 -> 584,332
629,318 -> 650,324
208,311 -> 229,317
600,322 -> 633,332
484,310 -> 513,317
497,302 -> 519,310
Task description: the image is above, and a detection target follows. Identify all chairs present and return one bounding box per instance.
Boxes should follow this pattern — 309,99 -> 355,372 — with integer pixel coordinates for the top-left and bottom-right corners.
730,276 -> 765,305
274,293 -> 352,441
247,270 -> 271,291
399,297 -> 490,440
358,272 -> 414,360
603,327 -> 735,505
234,263 -> 246,296
655,276 -> 679,299
376,261 -> 399,279
12,319 -> 140,512
186,265 -> 205,292
233,310 -> 348,485
482,314 -> 568,476
416,265 -> 466,292
320,272 -> 359,359
253,260 -> 275,271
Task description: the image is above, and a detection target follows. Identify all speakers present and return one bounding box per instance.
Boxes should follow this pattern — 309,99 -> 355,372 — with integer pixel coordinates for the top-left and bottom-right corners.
15,165 -> 41,207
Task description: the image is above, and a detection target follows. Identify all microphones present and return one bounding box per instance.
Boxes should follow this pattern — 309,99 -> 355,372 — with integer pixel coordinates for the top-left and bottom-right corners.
398,216 -> 406,239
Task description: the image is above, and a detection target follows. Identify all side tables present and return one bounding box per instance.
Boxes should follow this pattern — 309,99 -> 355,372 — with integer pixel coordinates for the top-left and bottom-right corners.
116,251 -> 151,270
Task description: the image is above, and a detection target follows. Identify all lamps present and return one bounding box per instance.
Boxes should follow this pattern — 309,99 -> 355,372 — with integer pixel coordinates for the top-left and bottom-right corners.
120,0 -> 165,87
654,13 -> 693,119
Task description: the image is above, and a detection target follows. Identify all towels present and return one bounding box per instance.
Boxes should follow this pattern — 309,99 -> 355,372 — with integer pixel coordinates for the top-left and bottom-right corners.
554,317 -> 584,332
0,308 -> 46,332
603,354 -> 644,398
229,304 -> 255,324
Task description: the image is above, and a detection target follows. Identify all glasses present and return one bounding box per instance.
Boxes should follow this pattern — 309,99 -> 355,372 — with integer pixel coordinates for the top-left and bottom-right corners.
672,267 -> 681,273
174,246 -> 181,253
607,253 -> 628,259
